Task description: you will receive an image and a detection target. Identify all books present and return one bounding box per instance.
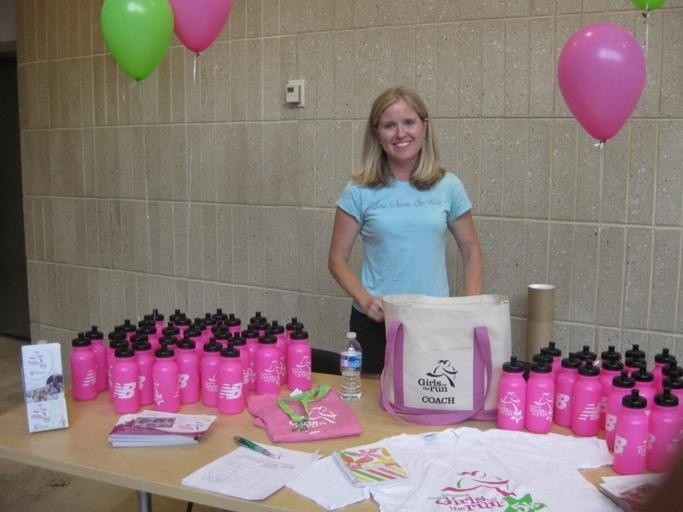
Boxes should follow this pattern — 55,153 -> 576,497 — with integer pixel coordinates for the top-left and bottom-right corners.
107,410 -> 218,448
599,472 -> 668,512
333,448 -> 409,487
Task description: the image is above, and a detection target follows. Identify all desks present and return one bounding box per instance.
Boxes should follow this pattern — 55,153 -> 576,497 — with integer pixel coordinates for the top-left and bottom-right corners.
0,372 -> 683,512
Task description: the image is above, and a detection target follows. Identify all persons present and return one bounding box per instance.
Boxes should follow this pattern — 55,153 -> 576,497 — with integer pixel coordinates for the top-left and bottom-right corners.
328,86 -> 485,373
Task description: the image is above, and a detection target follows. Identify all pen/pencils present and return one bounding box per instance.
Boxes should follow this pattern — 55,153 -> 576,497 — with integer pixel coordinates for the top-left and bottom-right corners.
233,435 -> 275,459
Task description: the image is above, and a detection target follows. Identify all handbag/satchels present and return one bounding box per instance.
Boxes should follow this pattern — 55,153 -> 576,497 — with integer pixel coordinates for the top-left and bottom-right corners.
379,294 -> 511,426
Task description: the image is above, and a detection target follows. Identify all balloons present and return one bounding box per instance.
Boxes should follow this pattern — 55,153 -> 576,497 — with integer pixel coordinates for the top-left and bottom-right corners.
100,0 -> 174,86
558,23 -> 646,150
631,0 -> 665,18
169,0 -> 232,59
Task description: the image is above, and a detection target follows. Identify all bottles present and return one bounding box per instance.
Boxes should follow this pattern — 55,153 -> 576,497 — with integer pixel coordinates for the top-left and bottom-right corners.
340,331 -> 363,401
497,342 -> 683,474
71,310 -> 312,413
